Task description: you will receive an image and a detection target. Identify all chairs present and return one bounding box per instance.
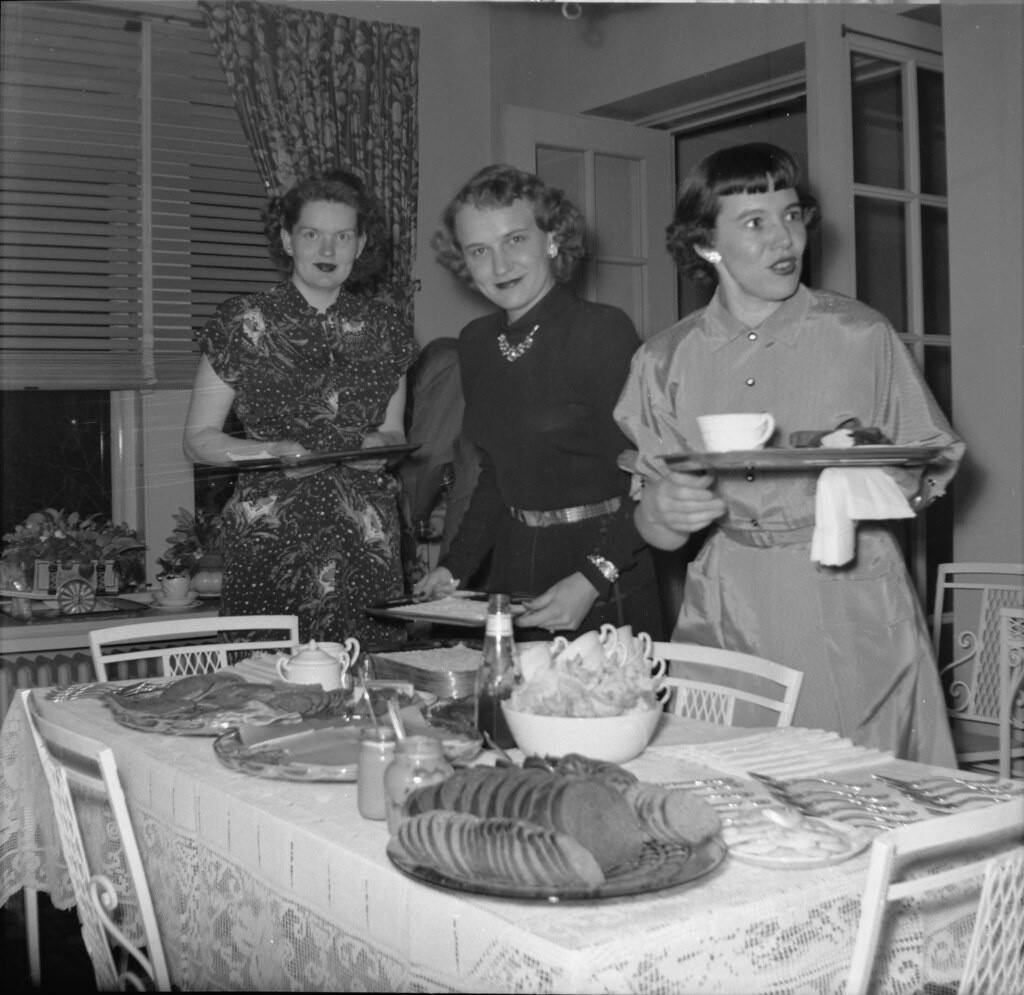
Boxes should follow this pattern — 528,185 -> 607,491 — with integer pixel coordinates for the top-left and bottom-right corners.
89,613 -> 299,682
933,561 -> 1024,783
845,795 -> 1023,995
644,640 -> 806,727
20,686 -> 172,993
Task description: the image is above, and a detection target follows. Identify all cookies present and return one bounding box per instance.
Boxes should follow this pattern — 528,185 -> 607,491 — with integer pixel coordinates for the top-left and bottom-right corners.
399,753 -> 719,890
721,805 -> 852,863
133,674 -> 412,719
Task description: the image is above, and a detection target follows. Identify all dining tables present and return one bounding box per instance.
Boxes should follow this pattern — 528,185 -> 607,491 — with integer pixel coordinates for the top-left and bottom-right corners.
0,676 -> 1024,995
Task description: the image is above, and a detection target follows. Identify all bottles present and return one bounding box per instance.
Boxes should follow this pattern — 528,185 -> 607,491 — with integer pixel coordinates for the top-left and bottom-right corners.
359,726 -> 396,820
474,594 -> 526,751
385,735 -> 454,836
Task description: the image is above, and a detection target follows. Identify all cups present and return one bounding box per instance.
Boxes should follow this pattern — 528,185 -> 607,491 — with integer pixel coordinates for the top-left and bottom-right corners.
698,413 -> 774,453
514,624 -> 671,716
277,639 -> 360,696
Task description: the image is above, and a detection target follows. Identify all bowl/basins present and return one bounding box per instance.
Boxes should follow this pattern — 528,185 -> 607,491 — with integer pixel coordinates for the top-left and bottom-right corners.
500,700 -> 663,764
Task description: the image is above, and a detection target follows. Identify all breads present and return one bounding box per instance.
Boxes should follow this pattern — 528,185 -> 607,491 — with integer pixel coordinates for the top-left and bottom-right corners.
789,426 -> 892,448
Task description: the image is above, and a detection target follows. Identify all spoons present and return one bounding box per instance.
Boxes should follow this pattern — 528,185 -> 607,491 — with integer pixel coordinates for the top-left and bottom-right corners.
46,681 -> 151,703
872,774 -> 1024,815
748,771 -> 924,831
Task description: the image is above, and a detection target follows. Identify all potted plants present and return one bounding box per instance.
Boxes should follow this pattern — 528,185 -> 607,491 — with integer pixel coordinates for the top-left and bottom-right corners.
165,505 -> 227,573
2,507 -> 149,595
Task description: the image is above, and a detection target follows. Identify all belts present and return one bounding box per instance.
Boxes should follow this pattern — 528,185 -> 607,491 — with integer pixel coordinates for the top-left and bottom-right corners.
507,493 -> 624,529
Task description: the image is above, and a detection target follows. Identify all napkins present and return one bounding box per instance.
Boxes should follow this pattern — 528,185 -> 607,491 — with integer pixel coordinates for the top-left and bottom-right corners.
647,726 -> 897,784
811,467 -> 914,566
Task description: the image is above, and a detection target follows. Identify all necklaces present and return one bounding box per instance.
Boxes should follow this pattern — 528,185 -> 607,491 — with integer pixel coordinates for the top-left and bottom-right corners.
497,325 -> 539,362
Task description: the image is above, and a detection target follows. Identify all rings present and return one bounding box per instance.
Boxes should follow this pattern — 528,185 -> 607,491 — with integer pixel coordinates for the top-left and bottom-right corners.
549,627 -> 554,633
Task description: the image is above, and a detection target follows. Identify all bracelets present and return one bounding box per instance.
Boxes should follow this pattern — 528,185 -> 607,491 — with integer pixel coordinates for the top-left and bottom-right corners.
588,555 -> 620,584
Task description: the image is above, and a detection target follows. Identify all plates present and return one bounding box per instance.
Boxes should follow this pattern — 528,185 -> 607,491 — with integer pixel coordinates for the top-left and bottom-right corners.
366,640 -> 483,700
725,817 -> 873,870
151,592 -> 203,611
353,686 -> 437,708
213,730 -> 483,782
109,698 -> 240,737
387,838 -> 728,898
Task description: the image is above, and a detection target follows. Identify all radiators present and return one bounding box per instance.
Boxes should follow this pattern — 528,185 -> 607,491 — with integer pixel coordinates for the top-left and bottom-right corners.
0,638 -> 220,726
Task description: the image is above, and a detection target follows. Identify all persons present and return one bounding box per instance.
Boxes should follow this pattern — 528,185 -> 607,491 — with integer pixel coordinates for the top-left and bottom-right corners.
609,143 -> 965,767
181,171 -> 423,656
411,162 -> 670,640
